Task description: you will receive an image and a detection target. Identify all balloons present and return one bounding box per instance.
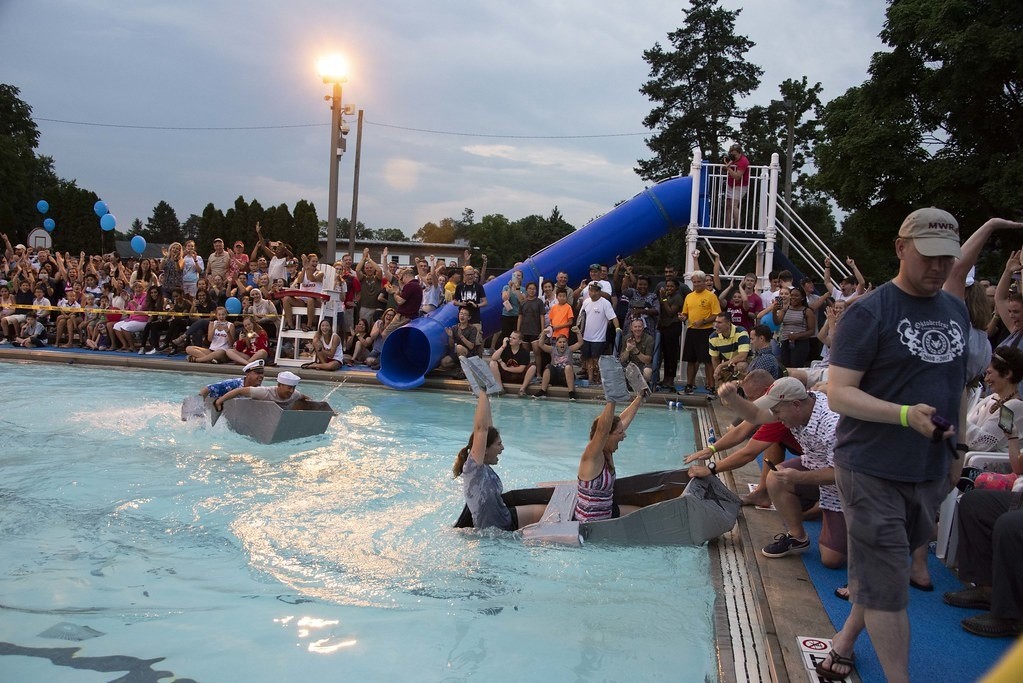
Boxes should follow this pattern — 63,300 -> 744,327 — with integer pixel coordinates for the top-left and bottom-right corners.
94,201 -> 108,217
225,297 -> 241,314
100,214 -> 116,231
44,218 -> 55,232
37,200 -> 49,214
131,236 -> 146,254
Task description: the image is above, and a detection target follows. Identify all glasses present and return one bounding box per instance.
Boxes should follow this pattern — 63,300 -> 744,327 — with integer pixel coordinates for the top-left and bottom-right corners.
286,261 -> 295,265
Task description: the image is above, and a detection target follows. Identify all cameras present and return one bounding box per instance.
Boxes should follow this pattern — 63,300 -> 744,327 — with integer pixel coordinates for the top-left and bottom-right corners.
932,415 -> 951,430
726,153 -> 735,163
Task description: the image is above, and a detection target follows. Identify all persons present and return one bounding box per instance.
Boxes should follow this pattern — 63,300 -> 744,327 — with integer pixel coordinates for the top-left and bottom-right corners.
718,377 -> 847,569
574,388 -> 644,523
834,217 -> 1023,599
198,359 -> 265,398
215,371 -> 311,411
965,345 -> 1023,474
0,220 -> 1023,438
974,423 -> 1023,492
944,489 -> 1023,637
452,386 -> 548,531
816,207 -> 970,683
683,368 -> 823,521
723,144 -> 749,228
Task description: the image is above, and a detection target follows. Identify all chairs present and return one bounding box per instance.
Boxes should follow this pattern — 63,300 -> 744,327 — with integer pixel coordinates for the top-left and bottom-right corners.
274,263 -> 341,367
936,449 -> 1023,559
945,454 -> 1012,568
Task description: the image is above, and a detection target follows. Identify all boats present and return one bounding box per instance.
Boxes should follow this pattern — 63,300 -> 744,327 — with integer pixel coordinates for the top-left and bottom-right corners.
452,467 -> 745,549
180,395 -> 340,445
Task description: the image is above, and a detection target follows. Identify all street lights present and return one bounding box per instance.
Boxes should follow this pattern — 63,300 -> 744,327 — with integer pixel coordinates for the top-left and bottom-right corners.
317,53 -> 352,266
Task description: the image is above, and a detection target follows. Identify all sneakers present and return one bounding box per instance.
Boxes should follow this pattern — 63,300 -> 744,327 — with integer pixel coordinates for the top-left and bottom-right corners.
568,391 -> 577,400
531,389 -> 547,398
761,532 -> 810,558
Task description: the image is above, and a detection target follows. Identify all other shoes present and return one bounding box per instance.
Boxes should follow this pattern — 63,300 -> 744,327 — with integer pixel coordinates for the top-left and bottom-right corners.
708,386 -> 717,398
518,390 -> 527,398
492,388 -> 507,396
577,366 -> 602,385
0,322 -> 383,372
961,611 -> 1023,638
944,585 -> 996,611
678,385 -> 694,395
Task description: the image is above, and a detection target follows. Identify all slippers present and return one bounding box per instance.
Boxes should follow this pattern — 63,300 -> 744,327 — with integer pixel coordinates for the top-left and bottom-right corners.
910,577 -> 934,592
815,650 -> 855,681
834,583 -> 850,601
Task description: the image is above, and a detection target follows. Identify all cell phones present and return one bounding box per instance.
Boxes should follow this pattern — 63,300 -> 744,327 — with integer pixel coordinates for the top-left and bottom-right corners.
39,274 -> 48,279
775,297 -> 784,310
242,329 -> 246,337
997,405 -> 1015,434
249,297 -> 254,306
273,279 -> 278,284
764,458 -> 777,472
512,272 -> 518,283
617,256 -> 622,262
218,323 -> 226,328
271,242 -> 279,246
584,279 -> 593,285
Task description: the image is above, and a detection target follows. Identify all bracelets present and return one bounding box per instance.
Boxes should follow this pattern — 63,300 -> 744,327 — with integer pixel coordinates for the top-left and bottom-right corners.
460,335 -> 464,338
356,334 -> 360,336
625,272 -> 630,275
635,351 -> 640,355
579,287 -> 583,290
318,348 -> 322,352
194,260 -> 198,262
226,330 -> 230,333
663,299 -> 667,301
516,288 -> 520,290
501,346 -> 505,349
174,259 -> 178,262
625,348 -> 629,352
213,285 -> 216,288
825,266 -> 830,268
718,378 -> 723,382
708,445 -> 716,452
369,259 -> 372,262
732,371 -> 739,377
703,319 -> 706,324
47,286 -> 50,288
1008,437 -> 1019,440
900,405 -> 909,426
957,443 -> 969,453
993,314 -> 1000,318
354,301 -> 358,305
28,271 -> 31,273
616,327 -> 620,329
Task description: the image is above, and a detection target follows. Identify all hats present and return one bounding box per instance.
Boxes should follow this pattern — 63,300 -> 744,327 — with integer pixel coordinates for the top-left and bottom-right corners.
277,370 -> 301,386
898,206 -> 961,260
842,275 -> 855,284
588,281 -> 603,288
213,238 -> 223,242
589,264 -> 602,272
284,258 -> 300,266
752,376 -> 808,410
242,359 -> 265,373
234,241 -> 245,248
15,244 -> 26,251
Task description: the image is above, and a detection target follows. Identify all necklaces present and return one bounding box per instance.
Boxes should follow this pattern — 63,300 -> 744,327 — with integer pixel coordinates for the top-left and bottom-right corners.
990,390 -> 1018,414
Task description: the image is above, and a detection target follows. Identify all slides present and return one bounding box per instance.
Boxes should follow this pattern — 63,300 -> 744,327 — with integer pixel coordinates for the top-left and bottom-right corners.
376,158 -> 710,389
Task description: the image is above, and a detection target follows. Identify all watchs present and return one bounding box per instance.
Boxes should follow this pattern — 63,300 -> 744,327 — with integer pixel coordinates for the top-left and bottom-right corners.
708,462 -> 717,475
